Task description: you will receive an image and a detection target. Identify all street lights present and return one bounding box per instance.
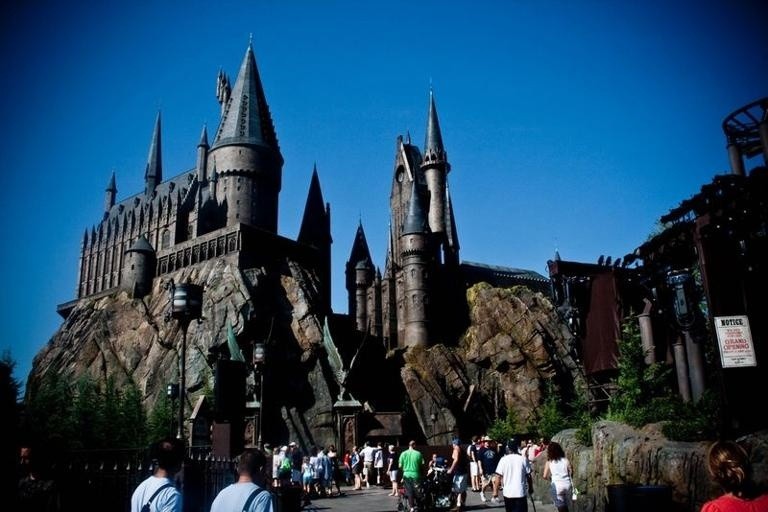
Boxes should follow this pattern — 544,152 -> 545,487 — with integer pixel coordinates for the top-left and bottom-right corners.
167,279 -> 204,448
165,382 -> 179,433
249,339 -> 275,453
429,412 -> 441,444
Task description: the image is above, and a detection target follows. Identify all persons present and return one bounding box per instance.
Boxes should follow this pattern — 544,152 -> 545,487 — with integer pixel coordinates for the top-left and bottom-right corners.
703,441 -> 768,511
264,435 -> 546,512
14,438 -> 66,511
210,449 -> 278,512
129,439 -> 185,511
543,442 -> 573,511
